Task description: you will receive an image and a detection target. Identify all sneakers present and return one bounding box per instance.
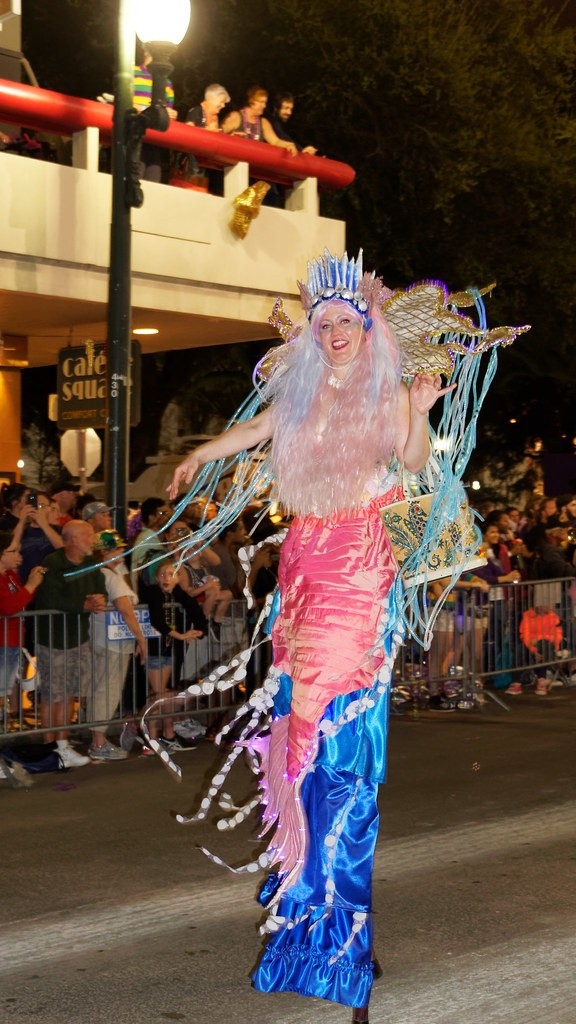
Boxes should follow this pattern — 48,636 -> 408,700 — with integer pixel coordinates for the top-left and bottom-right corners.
89,741 -> 130,761
120,720 -> 138,752
53,744 -> 90,768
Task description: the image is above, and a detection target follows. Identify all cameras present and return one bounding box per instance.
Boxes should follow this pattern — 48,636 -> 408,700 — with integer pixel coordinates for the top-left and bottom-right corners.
178,528 -> 189,537
38,567 -> 48,576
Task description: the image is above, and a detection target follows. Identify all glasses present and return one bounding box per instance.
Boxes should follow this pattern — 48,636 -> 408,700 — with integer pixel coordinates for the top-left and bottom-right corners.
4,550 -> 18,555
38,503 -> 50,509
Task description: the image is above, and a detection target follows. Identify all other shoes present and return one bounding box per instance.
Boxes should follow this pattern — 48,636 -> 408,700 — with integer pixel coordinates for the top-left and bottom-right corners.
142,739 -> 177,756
429,695 -> 457,712
183,719 -> 208,736
161,731 -> 197,751
0,765 -> 14,779
173,722 -> 200,738
505,682 -> 523,695
535,679 -> 548,696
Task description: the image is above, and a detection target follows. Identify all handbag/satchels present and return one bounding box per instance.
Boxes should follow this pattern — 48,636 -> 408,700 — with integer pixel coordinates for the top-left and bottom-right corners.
180,637 -> 214,683
378,455 -> 489,591
0,742 -> 63,773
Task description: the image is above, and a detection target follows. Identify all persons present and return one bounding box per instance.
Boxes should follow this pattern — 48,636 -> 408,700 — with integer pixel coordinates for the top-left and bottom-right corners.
0,482 -> 576,790
164,252 -> 458,1004
101,53 -> 319,210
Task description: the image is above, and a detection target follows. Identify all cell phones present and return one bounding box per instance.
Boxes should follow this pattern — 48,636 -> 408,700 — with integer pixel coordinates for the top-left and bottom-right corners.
26,493 -> 37,510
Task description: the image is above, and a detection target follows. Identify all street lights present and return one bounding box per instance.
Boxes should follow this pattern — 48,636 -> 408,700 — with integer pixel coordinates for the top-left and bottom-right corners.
97,0 -> 196,575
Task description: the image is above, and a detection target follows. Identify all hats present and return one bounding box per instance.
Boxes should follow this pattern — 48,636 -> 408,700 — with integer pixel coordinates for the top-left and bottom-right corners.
83,501 -> 116,520
94,529 -> 128,551
